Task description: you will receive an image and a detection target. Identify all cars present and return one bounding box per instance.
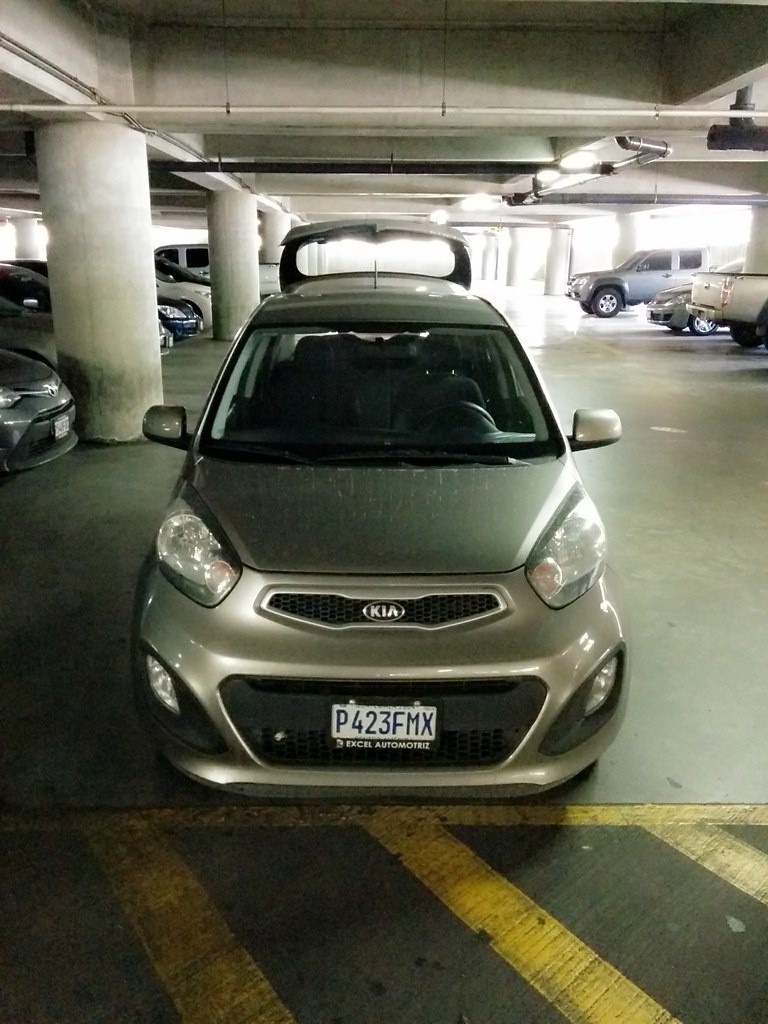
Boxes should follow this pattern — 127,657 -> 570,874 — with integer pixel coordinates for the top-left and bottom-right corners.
647,258 -> 745,336
129,219 -> 628,805
0,255 -> 212,476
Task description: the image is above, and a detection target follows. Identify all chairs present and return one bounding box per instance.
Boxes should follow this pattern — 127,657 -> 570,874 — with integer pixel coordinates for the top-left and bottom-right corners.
261,336 -> 363,430
391,333 -> 486,439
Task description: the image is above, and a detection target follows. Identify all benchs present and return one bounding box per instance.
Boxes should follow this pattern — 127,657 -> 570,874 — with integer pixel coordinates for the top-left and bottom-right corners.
339,333 -> 422,370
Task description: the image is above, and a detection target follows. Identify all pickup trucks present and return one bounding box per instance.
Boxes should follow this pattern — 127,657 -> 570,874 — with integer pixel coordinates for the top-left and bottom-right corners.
565,246 -> 708,318
685,273 -> 768,346
154,243 -> 280,294
